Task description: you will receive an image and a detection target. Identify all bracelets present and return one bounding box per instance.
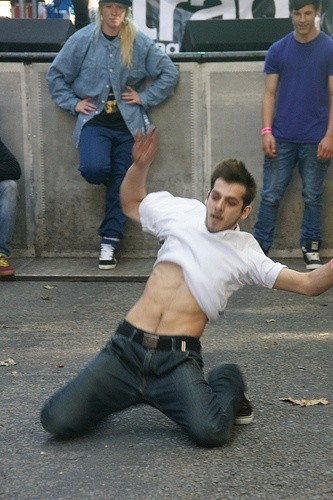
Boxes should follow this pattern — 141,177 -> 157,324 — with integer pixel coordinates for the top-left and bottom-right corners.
261,128 -> 271,135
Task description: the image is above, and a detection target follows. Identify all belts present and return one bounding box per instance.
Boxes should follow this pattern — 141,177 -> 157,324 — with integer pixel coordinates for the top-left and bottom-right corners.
115,320 -> 201,354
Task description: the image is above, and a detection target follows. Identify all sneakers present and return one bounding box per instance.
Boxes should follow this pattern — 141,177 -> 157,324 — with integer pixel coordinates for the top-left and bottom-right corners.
98,242 -> 118,270
235,389 -> 253,425
0,253 -> 15,276
302,240 -> 322,270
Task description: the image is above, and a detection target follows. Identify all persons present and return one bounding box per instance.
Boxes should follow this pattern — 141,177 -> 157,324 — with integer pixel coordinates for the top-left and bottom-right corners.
39,131 -> 332,447
46,0 -> 178,269
253,0 -> 333,268
0,139 -> 21,275
